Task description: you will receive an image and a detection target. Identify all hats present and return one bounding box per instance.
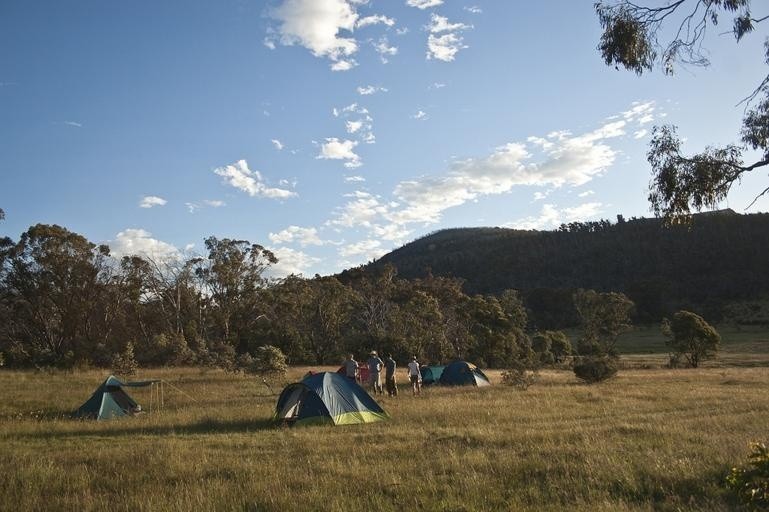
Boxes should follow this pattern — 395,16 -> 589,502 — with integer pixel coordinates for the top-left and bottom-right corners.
411,355 -> 417,360
369,351 -> 378,356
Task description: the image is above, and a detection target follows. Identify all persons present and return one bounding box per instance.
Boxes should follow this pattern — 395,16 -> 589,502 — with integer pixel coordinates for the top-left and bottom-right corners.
407,356 -> 423,395
367,350 -> 398,397
342,354 -> 358,382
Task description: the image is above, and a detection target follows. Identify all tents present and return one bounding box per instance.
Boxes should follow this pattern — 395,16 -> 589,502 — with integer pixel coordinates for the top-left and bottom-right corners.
277,371 -> 392,426
72,374 -> 161,421
337,364 -> 370,382
419,361 -> 490,388
